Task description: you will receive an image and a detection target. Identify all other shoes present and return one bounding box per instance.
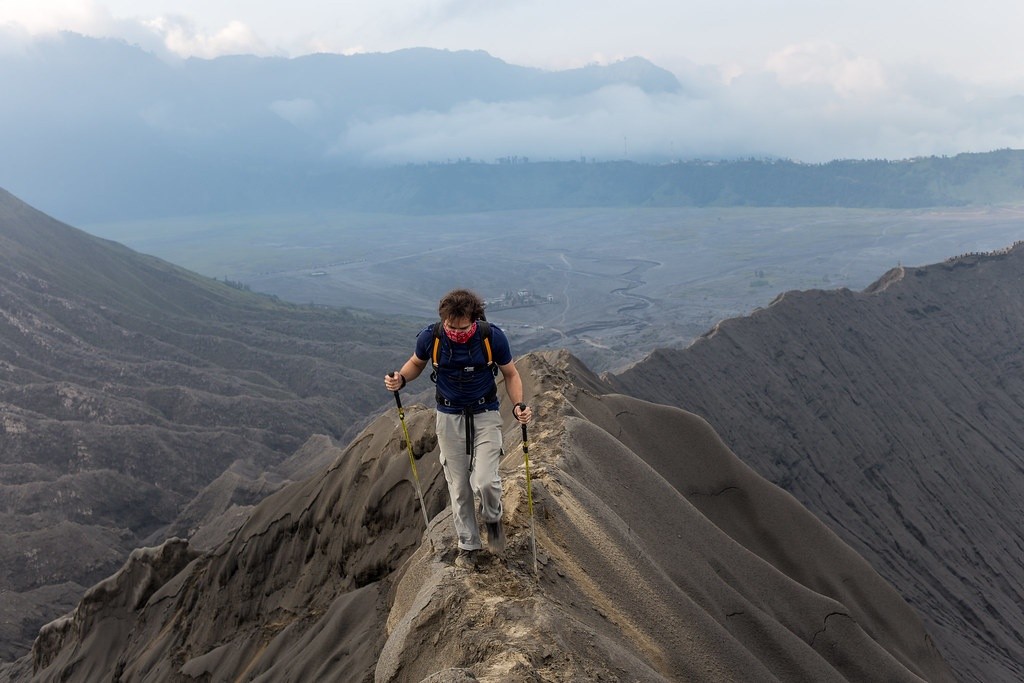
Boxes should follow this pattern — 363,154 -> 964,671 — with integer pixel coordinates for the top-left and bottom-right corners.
485,519 -> 505,554
455,549 -> 479,569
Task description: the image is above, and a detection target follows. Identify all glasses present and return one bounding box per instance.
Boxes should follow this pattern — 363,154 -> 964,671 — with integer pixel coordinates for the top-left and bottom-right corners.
446,356 -> 475,382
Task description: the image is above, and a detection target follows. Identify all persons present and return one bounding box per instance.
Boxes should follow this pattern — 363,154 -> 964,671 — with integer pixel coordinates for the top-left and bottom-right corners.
384,289 -> 532,572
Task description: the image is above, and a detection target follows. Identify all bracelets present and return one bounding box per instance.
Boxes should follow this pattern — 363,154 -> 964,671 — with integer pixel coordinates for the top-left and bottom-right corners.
396,374 -> 407,391
512,402 -> 523,422
